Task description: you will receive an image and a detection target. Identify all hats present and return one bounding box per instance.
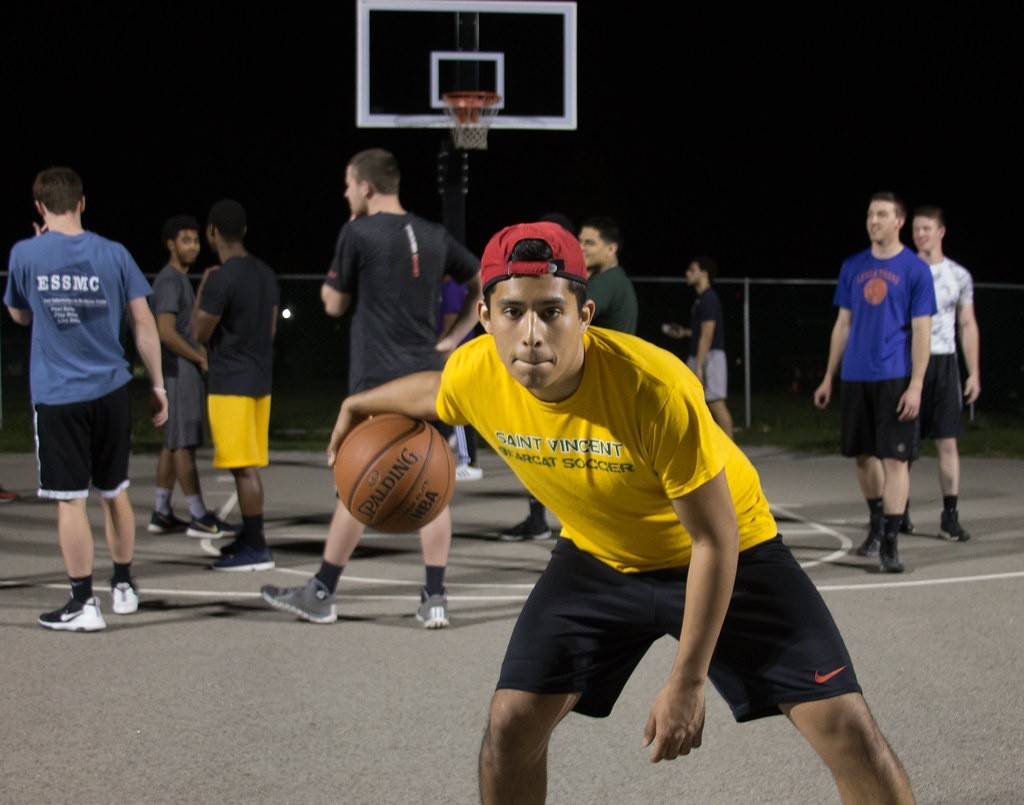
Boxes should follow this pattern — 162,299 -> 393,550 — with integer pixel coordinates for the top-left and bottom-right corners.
481,221 -> 589,295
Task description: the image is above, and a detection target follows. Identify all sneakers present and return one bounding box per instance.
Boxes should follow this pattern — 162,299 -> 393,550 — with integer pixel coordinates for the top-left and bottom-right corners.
499,515 -> 552,541
416,585 -> 450,628
903,511 -> 913,534
220,534 -> 245,558
941,511 -> 970,541
38,590 -> 106,632
456,463 -> 483,481
186,511 -> 242,539
211,544 -> 275,571
879,546 -> 905,573
261,577 -> 337,623
147,508 -> 191,534
109,576 -> 139,614
857,538 -> 879,556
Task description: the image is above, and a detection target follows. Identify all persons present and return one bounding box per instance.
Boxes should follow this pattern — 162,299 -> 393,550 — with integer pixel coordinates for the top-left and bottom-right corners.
328,222 -> 917,805
190,201 -> 276,573
260,149 -> 482,629
899,211 -> 979,541
501,219 -> 639,543
670,258 -> 733,437
814,191 -> 937,573
4,169 -> 169,631
440,277 -> 481,480
148,221 -> 234,539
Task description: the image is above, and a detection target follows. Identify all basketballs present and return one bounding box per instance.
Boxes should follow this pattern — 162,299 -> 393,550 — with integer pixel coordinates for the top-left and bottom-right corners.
332,413 -> 457,535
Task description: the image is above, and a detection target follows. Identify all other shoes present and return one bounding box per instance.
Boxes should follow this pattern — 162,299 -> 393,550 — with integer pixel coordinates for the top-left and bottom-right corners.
0,490 -> 21,503
